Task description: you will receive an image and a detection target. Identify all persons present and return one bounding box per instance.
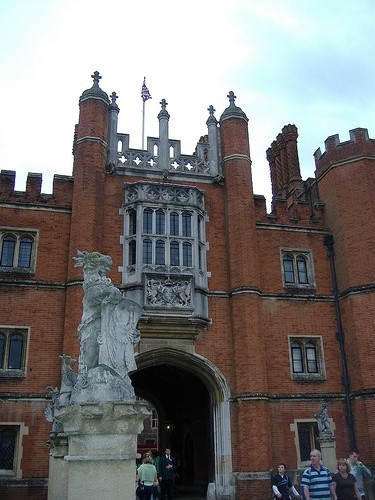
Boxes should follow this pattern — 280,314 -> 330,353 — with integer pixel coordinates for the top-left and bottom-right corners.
314,403 -> 330,432
271,463 -> 303,500
300,449 -> 337,500
158,447 -> 176,500
153,452 -> 161,479
332,460 -> 362,500
346,450 -> 372,500
136,456 -> 159,500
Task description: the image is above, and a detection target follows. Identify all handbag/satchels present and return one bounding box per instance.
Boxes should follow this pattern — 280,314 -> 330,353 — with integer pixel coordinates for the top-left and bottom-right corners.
136,481 -> 146,497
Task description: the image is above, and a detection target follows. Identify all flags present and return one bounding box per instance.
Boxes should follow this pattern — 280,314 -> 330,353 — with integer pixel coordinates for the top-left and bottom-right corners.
141,84 -> 152,99
